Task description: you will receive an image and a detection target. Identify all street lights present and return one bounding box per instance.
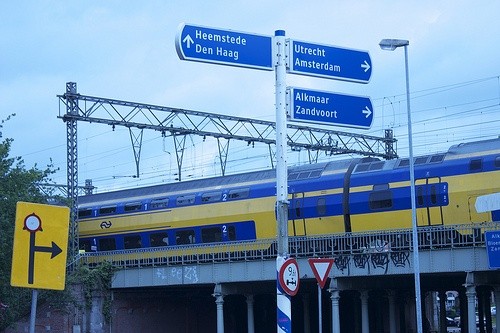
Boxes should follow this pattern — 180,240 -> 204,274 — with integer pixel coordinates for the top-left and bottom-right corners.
379,38 -> 423,333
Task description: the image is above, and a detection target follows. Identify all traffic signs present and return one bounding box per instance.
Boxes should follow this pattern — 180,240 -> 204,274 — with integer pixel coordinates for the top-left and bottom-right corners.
175,22 -> 376,131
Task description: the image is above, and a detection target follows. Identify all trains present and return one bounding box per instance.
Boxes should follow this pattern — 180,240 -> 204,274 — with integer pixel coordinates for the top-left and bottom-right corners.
48,135 -> 499,267
